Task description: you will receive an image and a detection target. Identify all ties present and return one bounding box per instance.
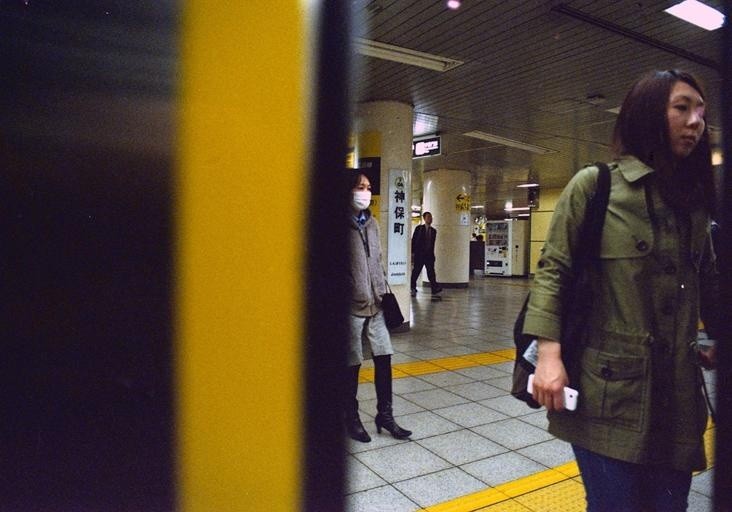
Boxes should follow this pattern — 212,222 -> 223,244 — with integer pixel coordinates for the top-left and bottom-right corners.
426,226 -> 431,237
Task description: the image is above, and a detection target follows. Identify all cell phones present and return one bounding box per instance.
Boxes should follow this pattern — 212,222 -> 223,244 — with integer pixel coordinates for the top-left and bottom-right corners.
527,374 -> 579,412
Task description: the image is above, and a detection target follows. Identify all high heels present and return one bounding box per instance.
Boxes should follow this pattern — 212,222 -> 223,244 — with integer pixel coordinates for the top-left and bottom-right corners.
375,409 -> 412,439
349,411 -> 371,442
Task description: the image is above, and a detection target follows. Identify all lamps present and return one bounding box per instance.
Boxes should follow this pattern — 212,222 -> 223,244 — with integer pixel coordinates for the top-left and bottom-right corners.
460,130 -> 561,156
349,36 -> 464,73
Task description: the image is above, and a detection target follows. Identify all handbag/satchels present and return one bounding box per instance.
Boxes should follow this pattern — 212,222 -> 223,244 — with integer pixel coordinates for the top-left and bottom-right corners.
509,284 -> 593,410
382,280 -> 404,330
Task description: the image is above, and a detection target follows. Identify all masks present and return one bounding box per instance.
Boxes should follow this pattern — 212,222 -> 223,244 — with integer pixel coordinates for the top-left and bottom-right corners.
350,191 -> 372,211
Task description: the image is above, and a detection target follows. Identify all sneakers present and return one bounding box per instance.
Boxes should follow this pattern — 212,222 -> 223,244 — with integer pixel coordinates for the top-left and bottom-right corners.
411,284 -> 443,296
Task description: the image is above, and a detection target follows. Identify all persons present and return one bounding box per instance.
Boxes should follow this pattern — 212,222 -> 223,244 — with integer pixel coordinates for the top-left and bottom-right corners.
507,69 -> 730,510
346,167 -> 412,442
410,211 -> 442,297
